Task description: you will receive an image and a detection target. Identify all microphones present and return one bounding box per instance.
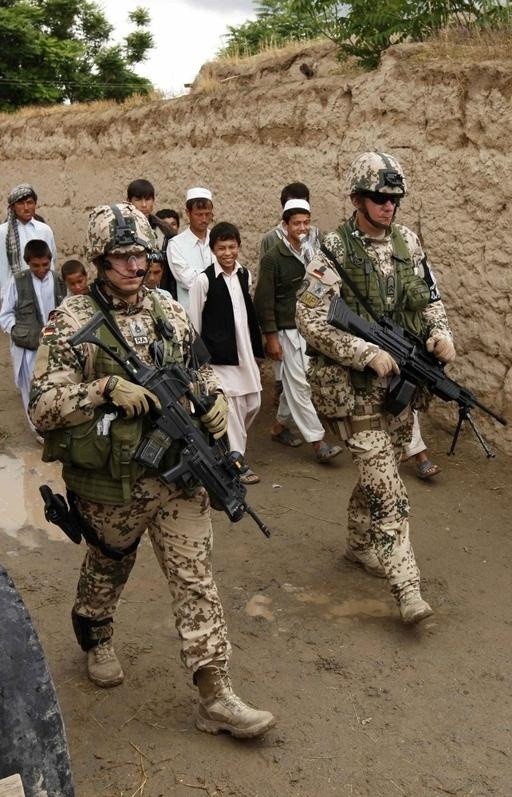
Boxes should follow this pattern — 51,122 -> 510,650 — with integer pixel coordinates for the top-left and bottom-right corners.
102,259 -> 146,278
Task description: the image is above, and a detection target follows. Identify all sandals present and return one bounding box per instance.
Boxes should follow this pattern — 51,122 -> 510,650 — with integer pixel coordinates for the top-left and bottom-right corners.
270,427 -> 303,448
315,443 -> 344,464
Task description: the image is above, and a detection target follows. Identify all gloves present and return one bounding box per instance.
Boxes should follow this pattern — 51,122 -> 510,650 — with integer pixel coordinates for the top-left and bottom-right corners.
425,331 -> 458,367
365,346 -> 402,381
198,387 -> 232,443
102,373 -> 162,421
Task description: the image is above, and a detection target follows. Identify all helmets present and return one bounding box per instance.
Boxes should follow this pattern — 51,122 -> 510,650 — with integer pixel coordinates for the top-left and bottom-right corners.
83,201 -> 156,263
345,150 -> 408,197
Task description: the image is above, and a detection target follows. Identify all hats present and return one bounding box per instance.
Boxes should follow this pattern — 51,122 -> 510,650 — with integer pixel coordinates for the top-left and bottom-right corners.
282,197 -> 312,218
184,186 -> 214,204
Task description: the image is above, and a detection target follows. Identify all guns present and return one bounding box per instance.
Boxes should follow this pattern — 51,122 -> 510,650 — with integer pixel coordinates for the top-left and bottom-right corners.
39,485 -> 81,544
65,312 -> 272,538
327,295 -> 507,423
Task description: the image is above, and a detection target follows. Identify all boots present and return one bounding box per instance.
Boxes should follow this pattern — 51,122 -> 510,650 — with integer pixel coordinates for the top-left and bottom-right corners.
192,662 -> 277,741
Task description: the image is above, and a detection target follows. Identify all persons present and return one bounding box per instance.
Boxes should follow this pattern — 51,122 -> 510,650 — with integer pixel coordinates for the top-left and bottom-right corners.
166,183 -> 262,486
26,202 -> 276,740
291,150 -> 458,627
127,177 -> 178,302
1,182 -> 88,445
399,415 -> 440,480
252,182 -> 345,463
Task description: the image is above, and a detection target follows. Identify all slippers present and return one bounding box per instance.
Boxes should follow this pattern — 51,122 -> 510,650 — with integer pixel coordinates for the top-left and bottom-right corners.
414,460 -> 442,480
239,468 -> 261,487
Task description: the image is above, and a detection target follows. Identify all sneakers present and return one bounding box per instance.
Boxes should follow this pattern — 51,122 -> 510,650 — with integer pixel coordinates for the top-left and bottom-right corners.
396,594 -> 436,629
342,541 -> 387,578
83,640 -> 125,689
